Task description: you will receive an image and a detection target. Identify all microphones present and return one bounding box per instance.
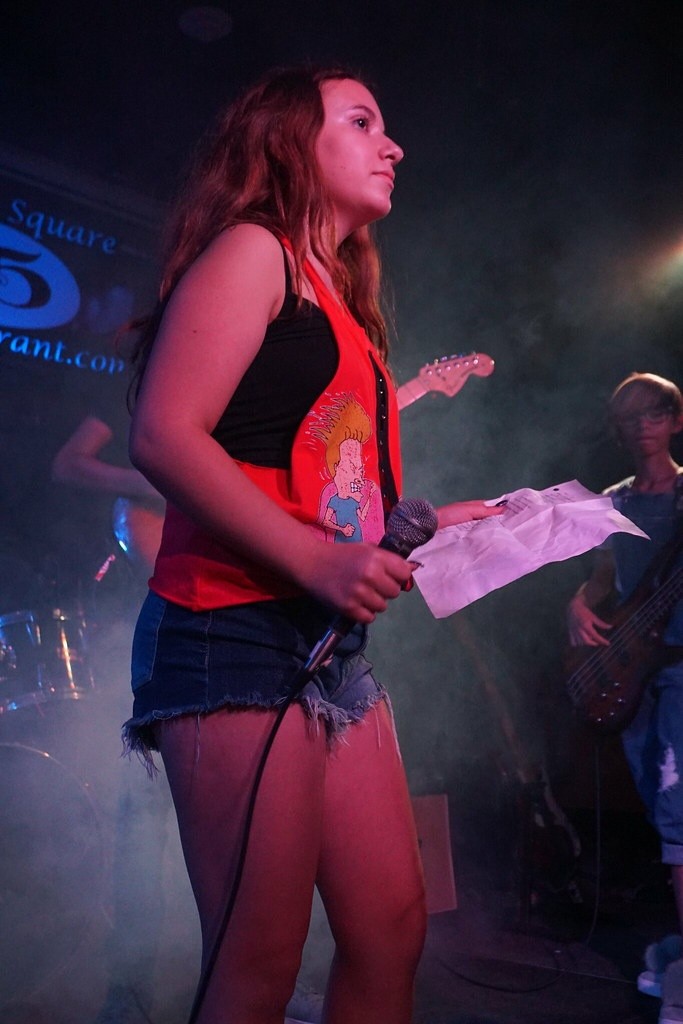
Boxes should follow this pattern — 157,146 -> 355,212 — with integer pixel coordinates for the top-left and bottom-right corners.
297,496 -> 438,691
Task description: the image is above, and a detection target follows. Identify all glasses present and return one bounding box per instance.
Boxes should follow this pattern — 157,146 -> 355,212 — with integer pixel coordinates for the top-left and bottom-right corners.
615,405 -> 676,428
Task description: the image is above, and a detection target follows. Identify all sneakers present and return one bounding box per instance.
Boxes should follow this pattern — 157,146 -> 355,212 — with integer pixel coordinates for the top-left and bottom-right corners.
637,934 -> 683,1024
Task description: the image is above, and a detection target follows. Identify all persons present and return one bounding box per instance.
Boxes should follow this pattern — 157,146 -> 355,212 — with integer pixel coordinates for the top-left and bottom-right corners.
560,371 -> 683,997
53,355 -> 165,503
119,61 -> 508,1022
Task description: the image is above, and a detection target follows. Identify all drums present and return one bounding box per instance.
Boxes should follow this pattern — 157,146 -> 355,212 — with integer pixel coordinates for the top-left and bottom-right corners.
0,608 -> 97,719
0,741 -> 113,1014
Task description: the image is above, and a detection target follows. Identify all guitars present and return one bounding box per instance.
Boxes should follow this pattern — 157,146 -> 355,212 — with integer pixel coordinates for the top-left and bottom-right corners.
112,349 -> 495,580
561,528 -> 683,735
449,612 -> 582,887
